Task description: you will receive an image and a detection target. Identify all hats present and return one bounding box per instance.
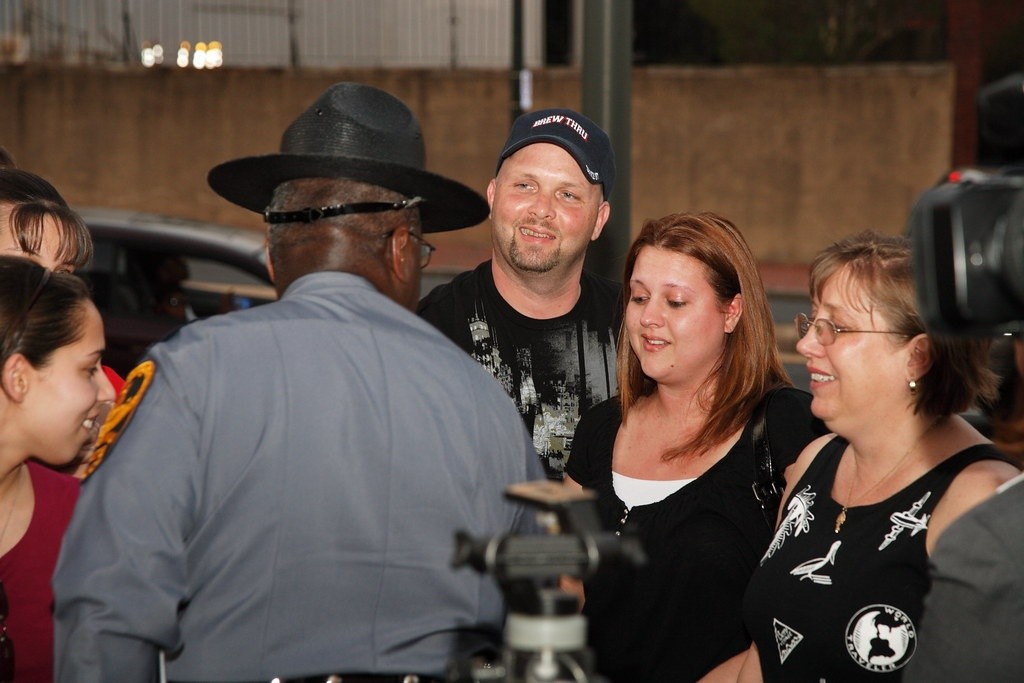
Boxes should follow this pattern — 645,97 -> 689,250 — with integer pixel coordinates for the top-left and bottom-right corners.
206,83 -> 491,234
495,107 -> 617,202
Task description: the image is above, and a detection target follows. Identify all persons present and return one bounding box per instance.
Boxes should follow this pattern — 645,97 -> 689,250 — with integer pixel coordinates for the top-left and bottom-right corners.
0,82 -> 1024,683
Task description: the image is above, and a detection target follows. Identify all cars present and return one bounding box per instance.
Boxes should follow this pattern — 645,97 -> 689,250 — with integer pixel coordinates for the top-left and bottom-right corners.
66,205 -> 468,319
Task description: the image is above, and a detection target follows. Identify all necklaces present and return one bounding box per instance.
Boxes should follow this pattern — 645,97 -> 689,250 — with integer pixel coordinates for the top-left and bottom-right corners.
834,441 -> 916,534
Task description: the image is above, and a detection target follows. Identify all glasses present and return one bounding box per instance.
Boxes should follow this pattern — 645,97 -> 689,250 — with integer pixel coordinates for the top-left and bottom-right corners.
381,228 -> 436,269
794,312 -> 920,347
